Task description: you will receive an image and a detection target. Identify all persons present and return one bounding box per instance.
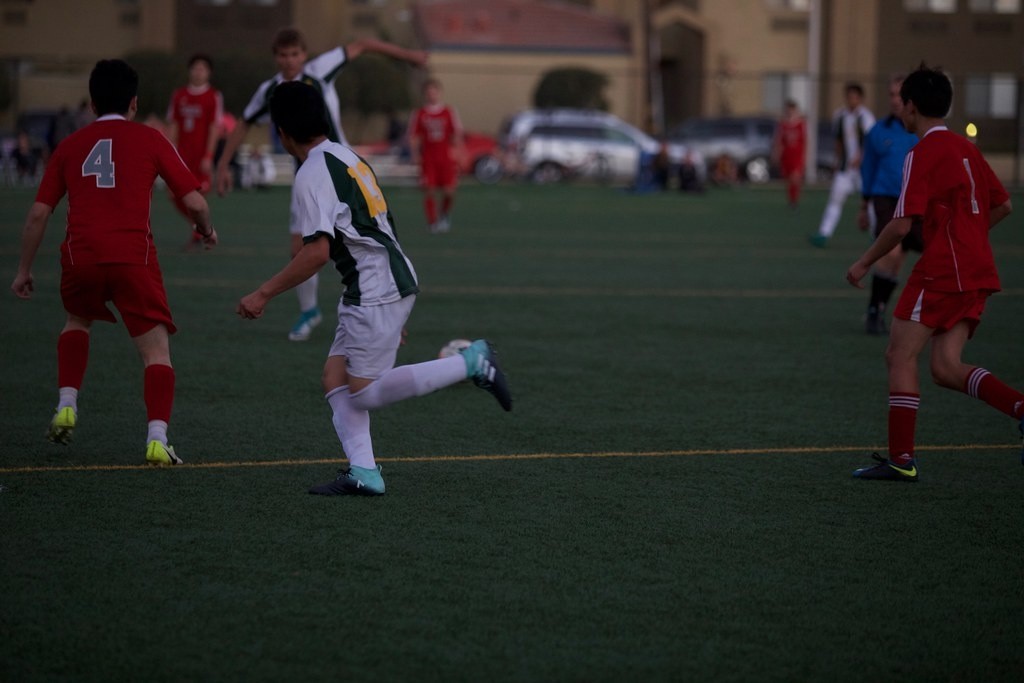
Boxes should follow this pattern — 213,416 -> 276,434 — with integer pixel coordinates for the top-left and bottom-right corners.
848,64 -> 1024,482
16,97 -> 276,196
407,80 -> 463,235
856,73 -> 929,337
236,82 -> 513,499
162,52 -> 223,248
213,28 -> 429,340
809,84 -> 877,248
769,98 -> 807,210
10,58 -> 219,465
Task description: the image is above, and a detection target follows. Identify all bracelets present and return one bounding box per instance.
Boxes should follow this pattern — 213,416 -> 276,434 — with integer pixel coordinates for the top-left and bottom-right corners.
204,226 -> 213,237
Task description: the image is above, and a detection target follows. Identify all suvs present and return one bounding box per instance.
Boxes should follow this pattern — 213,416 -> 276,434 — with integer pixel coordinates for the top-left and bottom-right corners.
497,105 -> 708,193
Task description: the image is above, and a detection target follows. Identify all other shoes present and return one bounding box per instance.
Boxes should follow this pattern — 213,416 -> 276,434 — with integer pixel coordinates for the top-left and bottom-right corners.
192,223 -> 203,240
810,233 -> 827,247
865,315 -> 889,333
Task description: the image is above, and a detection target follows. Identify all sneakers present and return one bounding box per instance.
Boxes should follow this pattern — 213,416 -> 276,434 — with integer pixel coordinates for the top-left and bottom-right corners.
853,453 -> 919,482
146,438 -> 183,466
464,337 -> 514,412
288,305 -> 322,342
310,463 -> 386,497
46,406 -> 77,446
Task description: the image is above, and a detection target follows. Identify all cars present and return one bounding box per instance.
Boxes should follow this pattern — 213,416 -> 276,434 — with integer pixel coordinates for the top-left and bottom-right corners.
650,115 -> 784,183
815,125 -> 846,181
392,128 -> 505,182
